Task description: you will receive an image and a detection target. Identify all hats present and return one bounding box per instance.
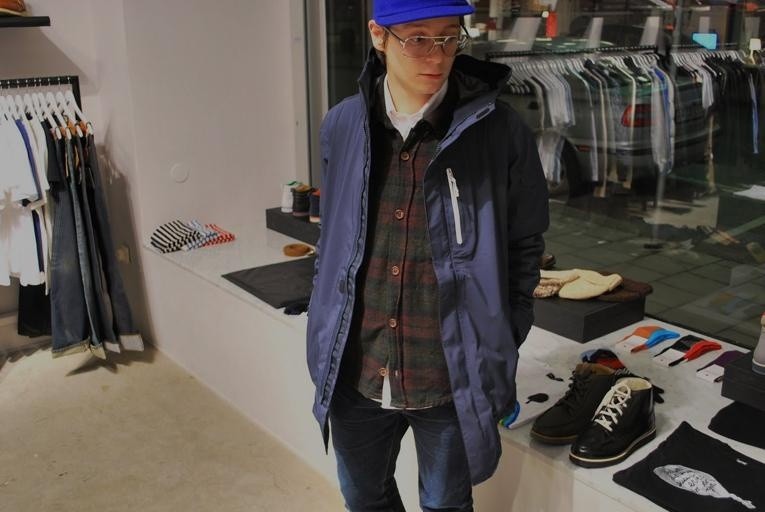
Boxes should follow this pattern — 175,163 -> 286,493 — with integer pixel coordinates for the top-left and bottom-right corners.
373,0 -> 474,26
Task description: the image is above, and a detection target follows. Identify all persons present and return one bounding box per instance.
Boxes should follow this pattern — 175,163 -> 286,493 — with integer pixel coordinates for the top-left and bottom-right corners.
306,0 -> 549,512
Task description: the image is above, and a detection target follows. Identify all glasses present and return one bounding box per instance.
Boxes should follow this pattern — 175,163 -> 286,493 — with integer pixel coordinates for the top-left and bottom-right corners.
382,23 -> 473,59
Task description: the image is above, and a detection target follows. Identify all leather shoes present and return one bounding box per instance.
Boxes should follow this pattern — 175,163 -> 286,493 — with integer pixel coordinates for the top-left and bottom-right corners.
541,251 -> 554,268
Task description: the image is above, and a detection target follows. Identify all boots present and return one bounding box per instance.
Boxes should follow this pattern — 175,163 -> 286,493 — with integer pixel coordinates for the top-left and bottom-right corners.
532,365 -> 616,446
569,376 -> 657,466
281,180 -> 320,222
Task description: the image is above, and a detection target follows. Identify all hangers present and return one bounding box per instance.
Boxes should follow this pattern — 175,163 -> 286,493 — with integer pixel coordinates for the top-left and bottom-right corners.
490,39 -> 751,94
0,74 -> 95,141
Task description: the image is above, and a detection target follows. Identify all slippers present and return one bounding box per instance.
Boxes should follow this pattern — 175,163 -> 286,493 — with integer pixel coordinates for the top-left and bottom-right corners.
532,268 -> 622,299
